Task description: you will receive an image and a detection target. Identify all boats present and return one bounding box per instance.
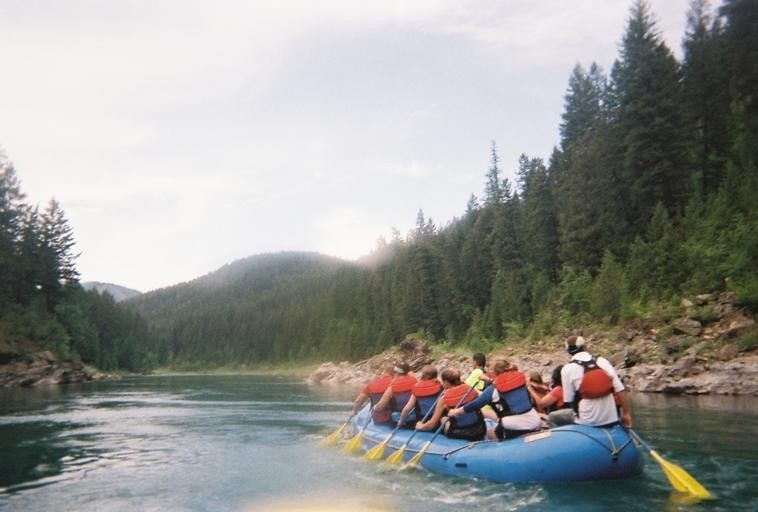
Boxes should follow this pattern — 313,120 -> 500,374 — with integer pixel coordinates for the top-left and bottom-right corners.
352,399 -> 646,485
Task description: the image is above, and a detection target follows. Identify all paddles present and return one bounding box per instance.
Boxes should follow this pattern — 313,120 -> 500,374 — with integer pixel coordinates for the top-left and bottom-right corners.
322,398 -> 371,446
344,410 -> 374,451
386,390 -> 443,464
626,429 -> 710,500
365,407 -> 416,461
406,377 -> 480,466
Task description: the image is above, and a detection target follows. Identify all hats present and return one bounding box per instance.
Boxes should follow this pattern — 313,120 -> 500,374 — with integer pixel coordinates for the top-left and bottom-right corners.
564,335 -> 584,354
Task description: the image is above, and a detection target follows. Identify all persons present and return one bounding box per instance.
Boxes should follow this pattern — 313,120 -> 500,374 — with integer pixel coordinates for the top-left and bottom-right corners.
350,336 -> 632,440
